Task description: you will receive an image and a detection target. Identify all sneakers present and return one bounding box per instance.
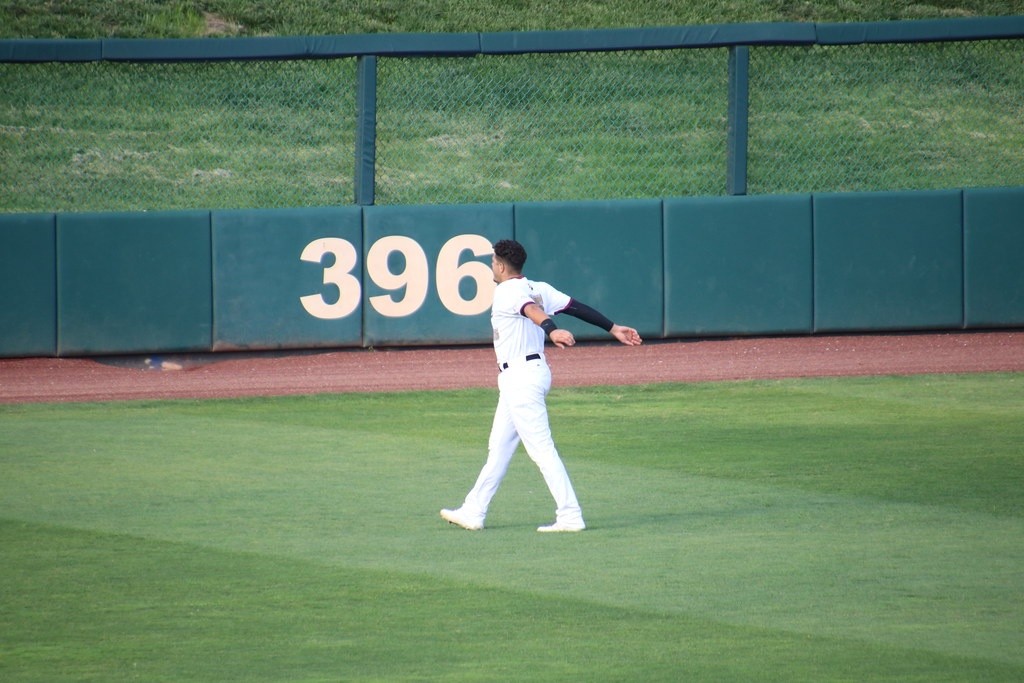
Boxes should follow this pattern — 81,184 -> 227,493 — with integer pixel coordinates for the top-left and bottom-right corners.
537,520 -> 585,532
440,508 -> 484,530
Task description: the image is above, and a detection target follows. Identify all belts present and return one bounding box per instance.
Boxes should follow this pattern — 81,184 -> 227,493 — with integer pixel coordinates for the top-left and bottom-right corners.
498,353 -> 541,372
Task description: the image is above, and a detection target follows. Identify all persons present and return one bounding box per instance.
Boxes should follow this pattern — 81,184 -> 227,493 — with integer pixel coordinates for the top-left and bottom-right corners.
439,239 -> 643,534
